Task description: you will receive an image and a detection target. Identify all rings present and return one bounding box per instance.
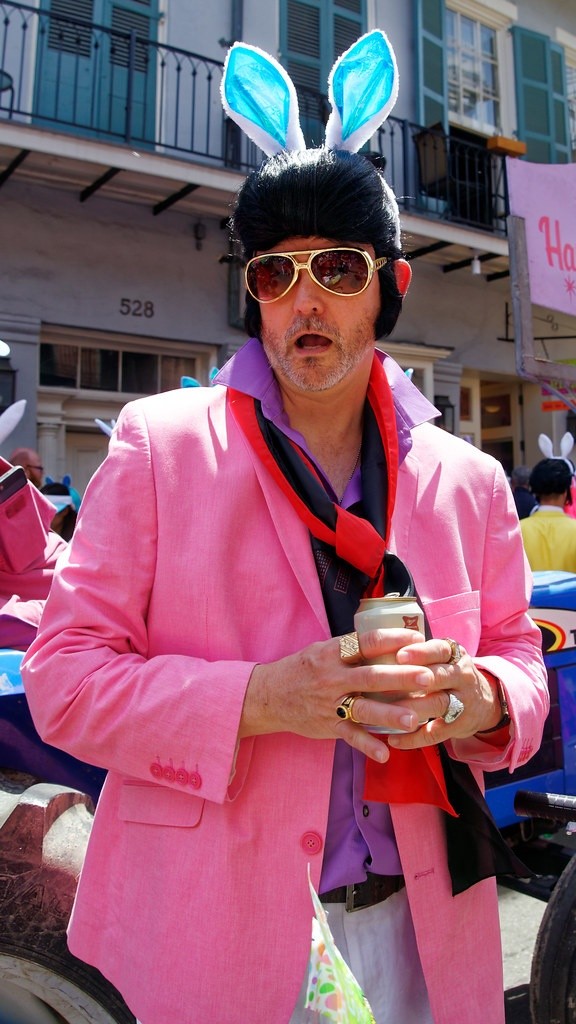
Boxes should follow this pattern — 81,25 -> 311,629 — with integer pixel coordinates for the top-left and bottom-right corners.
442,690 -> 464,723
336,696 -> 365,723
340,631 -> 366,664
445,639 -> 461,665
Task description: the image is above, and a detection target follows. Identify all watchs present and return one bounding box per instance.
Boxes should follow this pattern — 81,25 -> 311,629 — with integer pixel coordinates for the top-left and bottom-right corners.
478,669 -> 511,734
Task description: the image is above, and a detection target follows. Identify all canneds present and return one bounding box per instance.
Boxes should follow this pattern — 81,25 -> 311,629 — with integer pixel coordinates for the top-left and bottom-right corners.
354,592 -> 429,735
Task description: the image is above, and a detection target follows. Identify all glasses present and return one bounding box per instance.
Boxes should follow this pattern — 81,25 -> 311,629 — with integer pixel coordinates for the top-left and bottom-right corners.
244,246 -> 388,304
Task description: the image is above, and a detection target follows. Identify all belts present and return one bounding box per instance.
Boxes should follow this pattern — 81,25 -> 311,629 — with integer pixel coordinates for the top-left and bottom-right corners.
317,876 -> 412,913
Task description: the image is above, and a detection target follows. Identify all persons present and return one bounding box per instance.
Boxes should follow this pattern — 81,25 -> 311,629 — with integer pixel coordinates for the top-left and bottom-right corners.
19,29 -> 551,1024
509,432 -> 576,573
9,447 -> 82,542
